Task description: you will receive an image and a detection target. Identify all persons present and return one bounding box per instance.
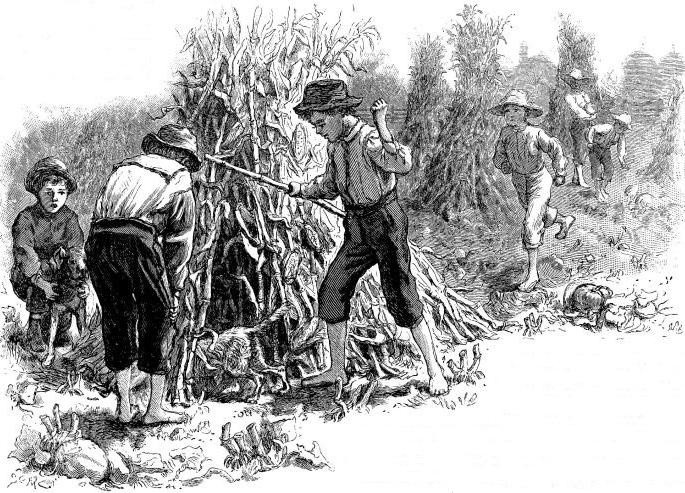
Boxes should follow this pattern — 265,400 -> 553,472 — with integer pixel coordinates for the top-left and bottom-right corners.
560,68 -> 597,187
288,79 -> 448,396
486,89 -> 577,292
586,114 -> 633,204
10,155 -> 89,353
84,124 -> 203,426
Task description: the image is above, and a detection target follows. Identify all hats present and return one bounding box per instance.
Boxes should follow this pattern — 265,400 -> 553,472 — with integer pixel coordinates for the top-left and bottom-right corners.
560,69 -> 591,85
611,113 -> 632,131
487,89 -> 544,119
293,79 -> 362,111
141,125 -> 201,173
24,158 -> 76,195
